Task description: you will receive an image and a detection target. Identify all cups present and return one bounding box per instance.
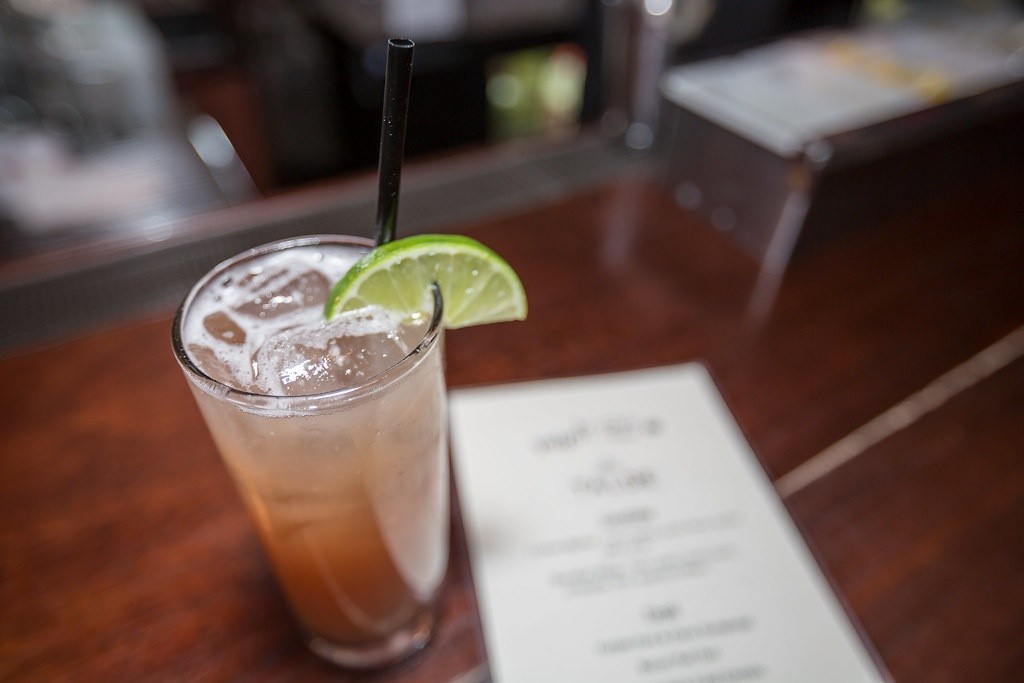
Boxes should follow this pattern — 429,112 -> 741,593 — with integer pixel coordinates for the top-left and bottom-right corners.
169,233 -> 450,670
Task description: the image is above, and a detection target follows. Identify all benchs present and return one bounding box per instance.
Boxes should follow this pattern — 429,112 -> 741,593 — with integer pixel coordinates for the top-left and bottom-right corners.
603,0 -> 1024,321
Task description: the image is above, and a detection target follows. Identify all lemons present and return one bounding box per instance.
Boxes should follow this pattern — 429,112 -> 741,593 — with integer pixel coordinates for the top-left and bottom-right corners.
325,233 -> 527,329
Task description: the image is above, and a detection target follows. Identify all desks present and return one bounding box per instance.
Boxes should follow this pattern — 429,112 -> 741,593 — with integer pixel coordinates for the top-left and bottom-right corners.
0,174 -> 1024,683
